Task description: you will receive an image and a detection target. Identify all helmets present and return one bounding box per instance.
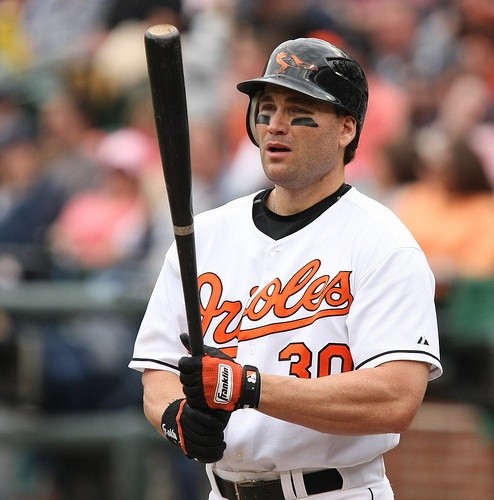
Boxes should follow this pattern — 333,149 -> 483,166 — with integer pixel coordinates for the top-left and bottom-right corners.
237,37 -> 369,127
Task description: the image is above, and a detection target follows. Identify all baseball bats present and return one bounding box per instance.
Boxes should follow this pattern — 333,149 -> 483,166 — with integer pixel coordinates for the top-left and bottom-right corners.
144,23 -> 205,360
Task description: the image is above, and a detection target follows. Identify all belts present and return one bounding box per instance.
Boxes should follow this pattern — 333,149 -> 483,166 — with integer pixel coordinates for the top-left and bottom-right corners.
213,468 -> 344,500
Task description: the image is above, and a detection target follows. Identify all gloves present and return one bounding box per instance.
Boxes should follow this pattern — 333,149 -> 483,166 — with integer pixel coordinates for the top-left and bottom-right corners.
179,333 -> 261,415
162,397 -> 231,464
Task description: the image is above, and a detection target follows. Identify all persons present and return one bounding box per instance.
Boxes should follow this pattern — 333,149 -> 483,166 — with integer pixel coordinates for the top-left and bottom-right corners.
0,0 -> 494,500
128,38 -> 443,500
